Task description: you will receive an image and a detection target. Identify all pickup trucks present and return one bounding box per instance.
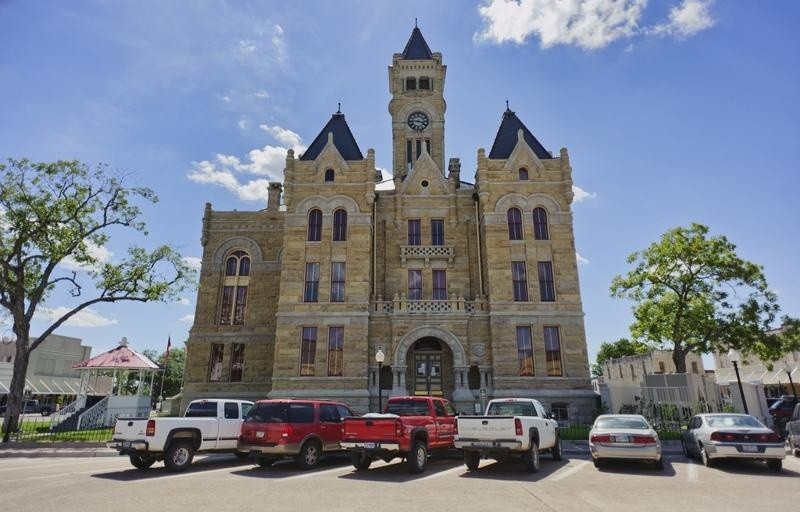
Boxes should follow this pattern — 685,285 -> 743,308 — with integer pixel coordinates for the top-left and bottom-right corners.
106,398 -> 283,473
453,397 -> 562,473
339,396 -> 466,475
0,399 -> 60,417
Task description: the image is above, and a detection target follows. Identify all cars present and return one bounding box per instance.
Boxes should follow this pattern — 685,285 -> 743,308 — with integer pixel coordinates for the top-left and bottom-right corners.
786,403 -> 800,456
680,412 -> 786,470
589,414 -> 662,468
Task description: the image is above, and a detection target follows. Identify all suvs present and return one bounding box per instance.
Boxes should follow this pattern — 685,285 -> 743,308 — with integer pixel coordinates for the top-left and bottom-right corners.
768,395 -> 800,423
237,396 -> 360,470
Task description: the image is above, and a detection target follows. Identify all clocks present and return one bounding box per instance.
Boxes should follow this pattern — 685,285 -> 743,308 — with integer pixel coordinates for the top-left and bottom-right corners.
406,109 -> 430,131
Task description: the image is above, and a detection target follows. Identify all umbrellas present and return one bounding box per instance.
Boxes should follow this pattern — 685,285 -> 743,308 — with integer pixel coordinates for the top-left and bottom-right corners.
70,344 -> 160,395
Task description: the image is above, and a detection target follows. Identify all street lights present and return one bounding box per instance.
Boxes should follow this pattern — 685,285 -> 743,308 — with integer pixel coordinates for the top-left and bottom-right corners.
783,360 -> 799,401
375,349 -> 384,414
728,349 -> 750,415
